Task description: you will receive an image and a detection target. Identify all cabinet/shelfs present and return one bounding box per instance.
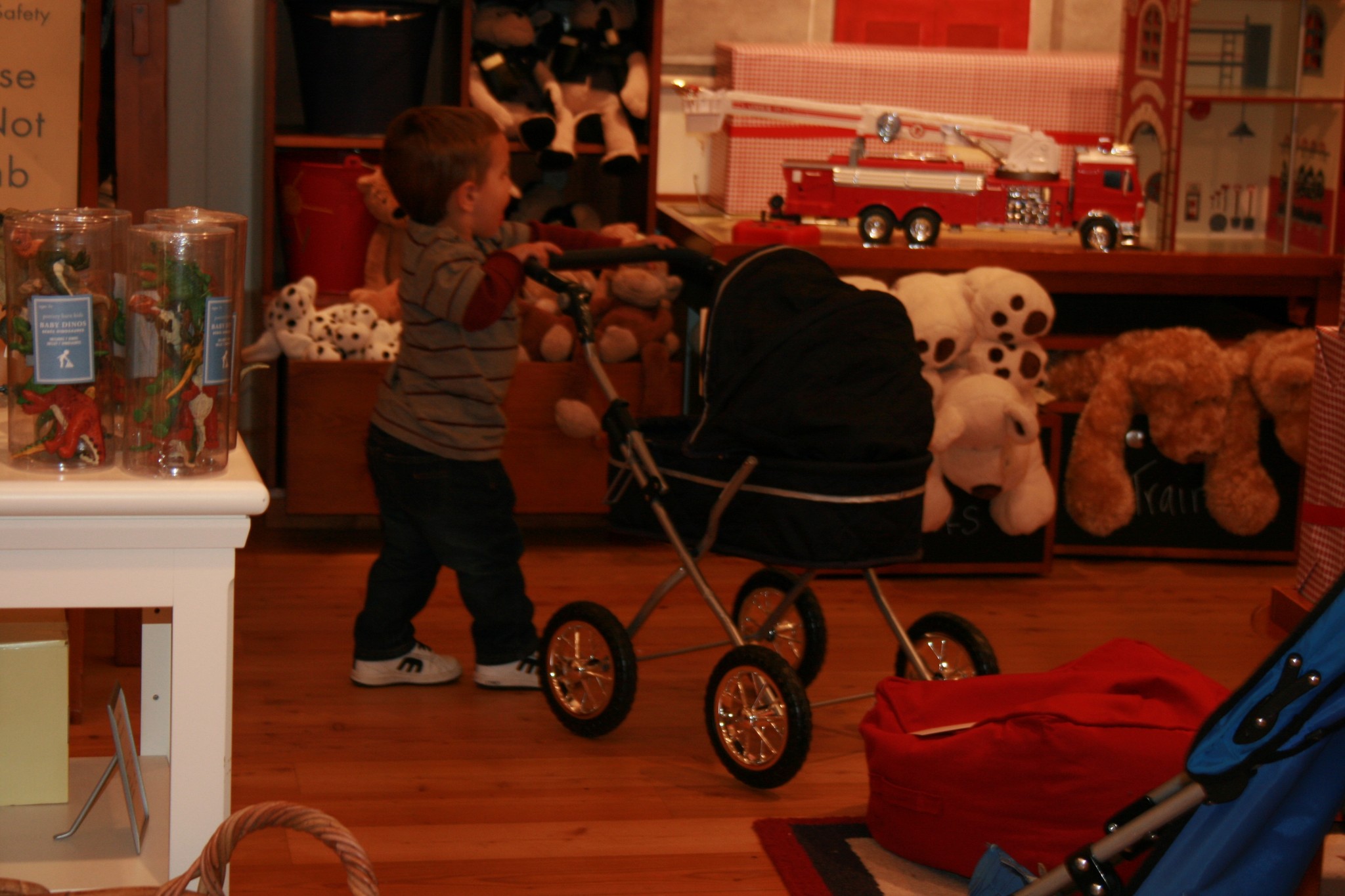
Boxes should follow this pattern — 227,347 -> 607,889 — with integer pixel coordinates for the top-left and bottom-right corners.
263,0 -> 666,541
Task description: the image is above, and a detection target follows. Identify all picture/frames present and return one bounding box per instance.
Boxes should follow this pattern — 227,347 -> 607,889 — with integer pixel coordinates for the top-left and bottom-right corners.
105,682 -> 150,855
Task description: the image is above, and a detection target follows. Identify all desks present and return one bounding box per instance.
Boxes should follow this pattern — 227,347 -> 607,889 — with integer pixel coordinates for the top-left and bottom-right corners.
654,200 -> 1345,351
0,431 -> 273,891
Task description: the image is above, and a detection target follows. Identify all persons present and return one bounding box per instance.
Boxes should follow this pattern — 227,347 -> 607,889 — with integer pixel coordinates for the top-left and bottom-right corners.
351,106 -> 678,690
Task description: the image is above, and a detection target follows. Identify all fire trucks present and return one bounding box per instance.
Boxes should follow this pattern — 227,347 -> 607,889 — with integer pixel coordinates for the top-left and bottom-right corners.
782,111 -> 1145,254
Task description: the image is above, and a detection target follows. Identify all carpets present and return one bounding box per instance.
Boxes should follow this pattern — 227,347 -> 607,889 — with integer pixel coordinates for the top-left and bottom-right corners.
754,813 -> 974,896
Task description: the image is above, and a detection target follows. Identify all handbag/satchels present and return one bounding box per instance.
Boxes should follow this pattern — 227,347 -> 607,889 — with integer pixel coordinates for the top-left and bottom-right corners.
858,634 -> 1227,880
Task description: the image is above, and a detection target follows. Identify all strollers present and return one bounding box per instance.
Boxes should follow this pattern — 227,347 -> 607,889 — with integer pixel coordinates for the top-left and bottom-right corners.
520,243 -> 999,791
963,567 -> 1344,895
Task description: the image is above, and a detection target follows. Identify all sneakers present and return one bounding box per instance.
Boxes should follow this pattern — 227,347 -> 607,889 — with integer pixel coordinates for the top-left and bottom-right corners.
347,642 -> 462,686
476,648 -> 542,687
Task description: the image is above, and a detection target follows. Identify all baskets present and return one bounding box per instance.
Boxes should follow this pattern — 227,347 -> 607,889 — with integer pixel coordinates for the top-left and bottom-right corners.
1,799 -> 384,896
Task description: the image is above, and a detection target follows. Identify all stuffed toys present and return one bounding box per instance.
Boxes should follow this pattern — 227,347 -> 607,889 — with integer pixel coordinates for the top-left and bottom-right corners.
837,266 -> 1057,536
1045,329 -> 1316,535
458,1 -> 688,360
230,160 -> 410,367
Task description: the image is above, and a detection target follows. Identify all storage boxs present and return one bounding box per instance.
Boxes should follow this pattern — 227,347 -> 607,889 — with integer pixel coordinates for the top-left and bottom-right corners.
766,411 -> 1064,573
1055,413 -> 1306,562
